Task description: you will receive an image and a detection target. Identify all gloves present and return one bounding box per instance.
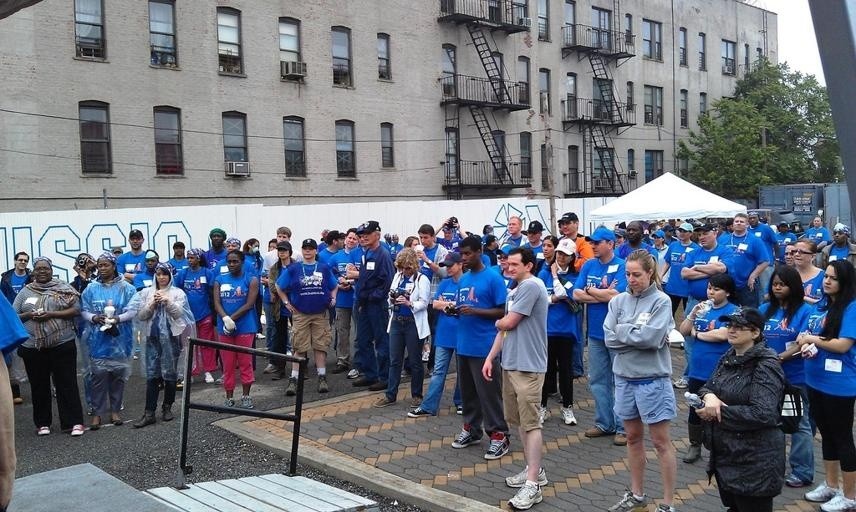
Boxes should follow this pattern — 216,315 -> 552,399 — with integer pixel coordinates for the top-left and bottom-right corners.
222,315 -> 237,335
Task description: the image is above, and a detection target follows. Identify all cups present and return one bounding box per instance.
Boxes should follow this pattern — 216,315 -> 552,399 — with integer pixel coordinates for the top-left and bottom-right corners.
415,245 -> 424,261
104,306 -> 115,319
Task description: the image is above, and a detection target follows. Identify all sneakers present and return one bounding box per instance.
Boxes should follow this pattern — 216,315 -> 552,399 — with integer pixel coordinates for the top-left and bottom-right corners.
804,481 -> 844,501
421,350 -> 430,362
256,333 -> 266,340
672,378 -> 689,388
821,493 -> 856,511
784,472 -> 806,487
408,397 -> 701,512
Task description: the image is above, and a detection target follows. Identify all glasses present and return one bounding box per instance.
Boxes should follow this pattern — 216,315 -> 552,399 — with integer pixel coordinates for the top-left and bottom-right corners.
791,248 -> 811,256
724,322 -> 748,332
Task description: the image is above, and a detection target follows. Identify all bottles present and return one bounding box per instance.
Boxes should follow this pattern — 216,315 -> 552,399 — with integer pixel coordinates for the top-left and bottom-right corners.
696,298 -> 715,319
566,298 -> 583,313
684,392 -> 705,409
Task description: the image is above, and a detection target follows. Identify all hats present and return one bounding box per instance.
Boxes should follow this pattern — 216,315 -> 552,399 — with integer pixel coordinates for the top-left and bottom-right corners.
277,221 -> 381,250
719,306 -> 765,331
439,252 -> 463,266
130,230 -> 143,239
496,213 -> 719,256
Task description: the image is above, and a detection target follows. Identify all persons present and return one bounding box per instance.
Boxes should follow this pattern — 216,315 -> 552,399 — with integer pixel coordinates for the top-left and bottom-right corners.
68,220 -> 402,432
12,256 -> 85,437
3,353 -> 23,405
0,288 -> 30,512
0,252 -> 35,307
372,213 -> 856,512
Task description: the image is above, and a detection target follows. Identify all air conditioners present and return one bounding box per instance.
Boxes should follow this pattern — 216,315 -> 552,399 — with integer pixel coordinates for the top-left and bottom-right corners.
225,162 -> 250,176
519,18 -> 533,28
282,61 -> 307,80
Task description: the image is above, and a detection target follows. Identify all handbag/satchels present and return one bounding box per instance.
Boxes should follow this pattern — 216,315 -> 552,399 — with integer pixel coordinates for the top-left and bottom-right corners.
779,384 -> 808,433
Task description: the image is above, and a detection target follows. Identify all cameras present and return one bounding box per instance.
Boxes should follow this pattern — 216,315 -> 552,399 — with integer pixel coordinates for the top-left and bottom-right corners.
445,305 -> 460,318
391,287 -> 410,302
